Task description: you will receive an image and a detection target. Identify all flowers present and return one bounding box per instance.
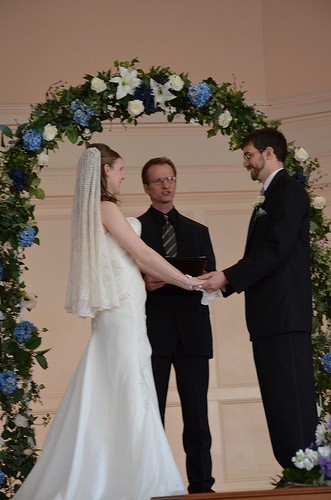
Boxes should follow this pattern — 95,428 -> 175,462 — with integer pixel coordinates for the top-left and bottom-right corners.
0,57 -> 330,499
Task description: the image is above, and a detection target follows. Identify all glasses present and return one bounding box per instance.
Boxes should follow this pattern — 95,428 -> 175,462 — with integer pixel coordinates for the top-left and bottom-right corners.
150,176 -> 176,184
243,150 -> 263,160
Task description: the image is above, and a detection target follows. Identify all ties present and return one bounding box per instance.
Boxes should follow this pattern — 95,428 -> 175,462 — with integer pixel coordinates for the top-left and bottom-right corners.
161,215 -> 178,257
259,186 -> 264,197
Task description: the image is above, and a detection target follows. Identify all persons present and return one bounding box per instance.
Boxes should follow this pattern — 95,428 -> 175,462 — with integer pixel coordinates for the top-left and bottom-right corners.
199,127 -> 319,488
135,157 -> 216,495
13,142 -> 225,500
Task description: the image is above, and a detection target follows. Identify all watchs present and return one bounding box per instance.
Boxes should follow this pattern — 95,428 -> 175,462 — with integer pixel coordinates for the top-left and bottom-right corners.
185,274 -> 193,289
